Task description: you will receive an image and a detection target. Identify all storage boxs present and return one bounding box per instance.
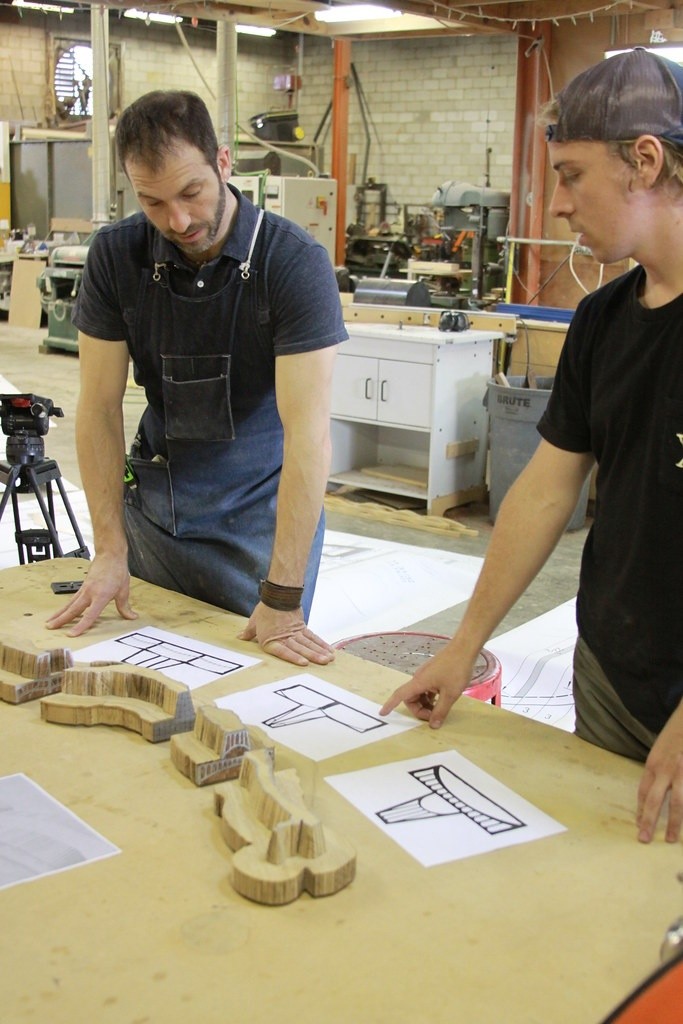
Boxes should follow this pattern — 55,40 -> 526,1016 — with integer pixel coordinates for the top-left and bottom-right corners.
251,111 -> 302,142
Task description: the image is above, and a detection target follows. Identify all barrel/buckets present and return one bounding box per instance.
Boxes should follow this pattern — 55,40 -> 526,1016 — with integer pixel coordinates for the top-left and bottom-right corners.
487,372 -> 592,533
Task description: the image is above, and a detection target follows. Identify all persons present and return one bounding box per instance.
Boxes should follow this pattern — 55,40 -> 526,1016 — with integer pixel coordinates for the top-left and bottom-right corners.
380,48 -> 682,844
44,90 -> 334,667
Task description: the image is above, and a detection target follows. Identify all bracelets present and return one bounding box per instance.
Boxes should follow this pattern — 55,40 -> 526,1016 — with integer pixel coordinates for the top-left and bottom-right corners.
261,581 -> 303,611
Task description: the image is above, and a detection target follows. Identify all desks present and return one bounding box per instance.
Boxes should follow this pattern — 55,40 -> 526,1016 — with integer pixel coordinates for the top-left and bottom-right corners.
0,556 -> 683,1024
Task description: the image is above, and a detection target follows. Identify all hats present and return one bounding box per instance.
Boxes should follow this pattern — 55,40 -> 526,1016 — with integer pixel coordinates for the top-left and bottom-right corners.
546,47 -> 683,141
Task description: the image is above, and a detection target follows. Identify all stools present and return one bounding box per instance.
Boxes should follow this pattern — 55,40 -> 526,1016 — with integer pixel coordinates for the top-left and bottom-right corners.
331,631 -> 502,708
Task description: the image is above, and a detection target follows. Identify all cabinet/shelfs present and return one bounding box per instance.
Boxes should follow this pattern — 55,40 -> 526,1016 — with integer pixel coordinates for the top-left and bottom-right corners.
326,322 -> 506,516
